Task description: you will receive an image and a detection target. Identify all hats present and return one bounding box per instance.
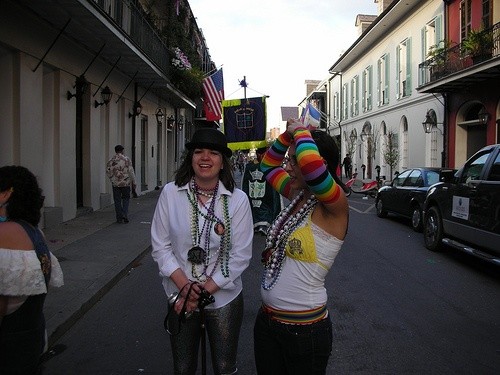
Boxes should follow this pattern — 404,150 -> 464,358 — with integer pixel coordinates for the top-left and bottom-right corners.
185,128 -> 232,158
258,147 -> 266,154
115,145 -> 124,152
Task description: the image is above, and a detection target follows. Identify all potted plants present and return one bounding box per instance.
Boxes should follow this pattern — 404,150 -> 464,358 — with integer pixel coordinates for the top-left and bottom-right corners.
459,28 -> 491,67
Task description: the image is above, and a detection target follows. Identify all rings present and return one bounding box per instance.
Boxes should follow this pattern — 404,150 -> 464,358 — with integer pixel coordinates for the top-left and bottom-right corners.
185,312 -> 188,314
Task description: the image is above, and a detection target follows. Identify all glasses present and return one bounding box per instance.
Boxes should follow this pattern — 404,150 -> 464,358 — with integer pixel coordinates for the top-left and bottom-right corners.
287,154 -> 297,165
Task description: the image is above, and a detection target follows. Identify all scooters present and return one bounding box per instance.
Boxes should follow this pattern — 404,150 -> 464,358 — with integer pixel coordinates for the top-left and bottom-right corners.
344,168 -> 379,197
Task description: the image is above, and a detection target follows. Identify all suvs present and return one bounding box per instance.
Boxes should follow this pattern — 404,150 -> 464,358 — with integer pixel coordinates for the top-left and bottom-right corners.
422,144 -> 500,263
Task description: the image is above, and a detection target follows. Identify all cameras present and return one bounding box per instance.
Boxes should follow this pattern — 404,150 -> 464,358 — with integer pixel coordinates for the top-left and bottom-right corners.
191,284 -> 215,312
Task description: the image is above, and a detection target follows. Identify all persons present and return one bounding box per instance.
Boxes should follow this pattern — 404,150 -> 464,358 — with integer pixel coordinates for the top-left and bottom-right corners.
231,154 -> 247,177
253,120 -> 349,375
241,148 -> 284,234
0,165 -> 65,375
343,154 -> 351,178
106,145 -> 136,223
151,129 -> 254,375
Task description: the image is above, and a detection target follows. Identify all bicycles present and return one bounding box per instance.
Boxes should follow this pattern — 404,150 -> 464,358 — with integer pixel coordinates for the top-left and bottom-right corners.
239,164 -> 244,175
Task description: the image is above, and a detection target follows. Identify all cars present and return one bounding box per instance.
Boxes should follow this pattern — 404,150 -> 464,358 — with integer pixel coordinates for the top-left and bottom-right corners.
374,167 -> 457,232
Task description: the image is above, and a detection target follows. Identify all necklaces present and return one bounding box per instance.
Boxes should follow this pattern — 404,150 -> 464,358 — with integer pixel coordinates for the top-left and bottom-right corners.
186,177 -> 229,282
261,190 -> 319,291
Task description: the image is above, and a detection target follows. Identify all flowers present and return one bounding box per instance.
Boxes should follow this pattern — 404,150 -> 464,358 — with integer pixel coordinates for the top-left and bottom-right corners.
173,46 -> 192,72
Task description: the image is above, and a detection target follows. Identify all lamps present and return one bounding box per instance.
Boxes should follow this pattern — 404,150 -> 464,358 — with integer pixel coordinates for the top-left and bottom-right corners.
67,74 -> 89,100
422,113 -> 445,133
153,109 -> 164,124
359,127 -> 374,141
350,130 -> 358,144
477,106 -> 492,127
94,86 -> 115,108
167,114 -> 175,127
129,98 -> 143,118
179,118 -> 184,130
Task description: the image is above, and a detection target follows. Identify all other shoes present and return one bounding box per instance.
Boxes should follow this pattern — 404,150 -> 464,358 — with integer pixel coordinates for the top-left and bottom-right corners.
254,229 -> 258,232
121,215 -> 129,222
259,228 -> 268,235
117,220 -> 122,223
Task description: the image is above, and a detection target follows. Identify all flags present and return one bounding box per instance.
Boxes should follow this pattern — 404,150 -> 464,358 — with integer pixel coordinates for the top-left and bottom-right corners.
202,94 -> 222,120
202,69 -> 224,116
299,104 -> 320,130
